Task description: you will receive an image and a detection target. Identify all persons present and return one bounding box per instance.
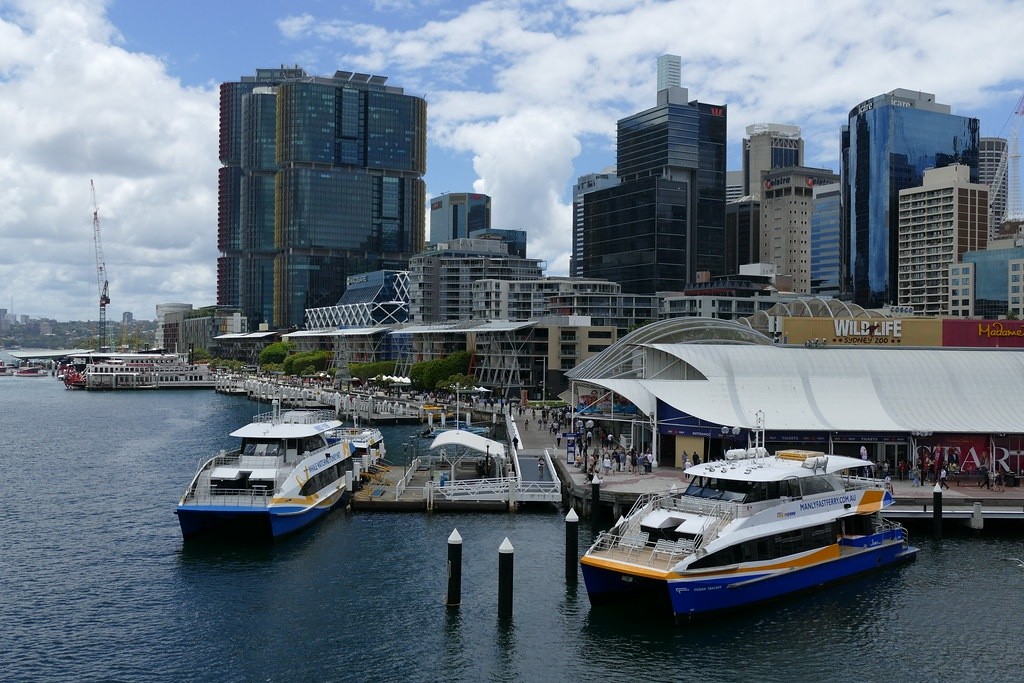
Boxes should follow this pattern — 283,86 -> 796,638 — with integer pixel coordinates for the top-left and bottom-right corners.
512,436 -> 518,452
577,440 -> 588,457
681,450 -> 700,479
601,431 -> 614,450
440,475 -> 445,486
898,461 -> 905,483
912,465 -> 920,487
538,456 -> 545,467
532,409 -> 536,420
583,444 -> 603,485
885,474 -> 894,495
409,390 -> 455,400
879,460 -> 888,478
940,468 -> 949,490
524,418 -> 529,431
538,403 -> 571,435
555,429 -> 562,447
573,454 -> 580,467
587,430 -> 592,447
980,470 -> 1005,493
483,398 -> 506,412
603,444 -> 654,476
521,405 -> 526,414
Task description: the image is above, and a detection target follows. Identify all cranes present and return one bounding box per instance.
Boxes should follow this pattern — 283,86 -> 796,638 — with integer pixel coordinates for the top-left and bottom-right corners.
88,178 -> 112,354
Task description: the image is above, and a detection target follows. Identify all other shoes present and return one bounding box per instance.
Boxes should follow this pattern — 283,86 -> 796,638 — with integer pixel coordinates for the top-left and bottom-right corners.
991,487 -> 1004,491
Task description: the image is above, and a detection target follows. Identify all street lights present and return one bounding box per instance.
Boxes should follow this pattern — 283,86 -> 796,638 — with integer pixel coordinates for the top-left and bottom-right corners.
575,418 -> 594,472
455,381 -> 461,430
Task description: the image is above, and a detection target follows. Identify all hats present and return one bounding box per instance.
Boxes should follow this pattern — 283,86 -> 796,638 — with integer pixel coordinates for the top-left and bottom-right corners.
687,459 -> 690,461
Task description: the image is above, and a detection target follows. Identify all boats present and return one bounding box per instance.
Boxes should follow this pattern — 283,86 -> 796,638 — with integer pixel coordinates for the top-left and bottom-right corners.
0,366 -> 48,377
169,390 -> 388,546
577,441 -> 922,622
